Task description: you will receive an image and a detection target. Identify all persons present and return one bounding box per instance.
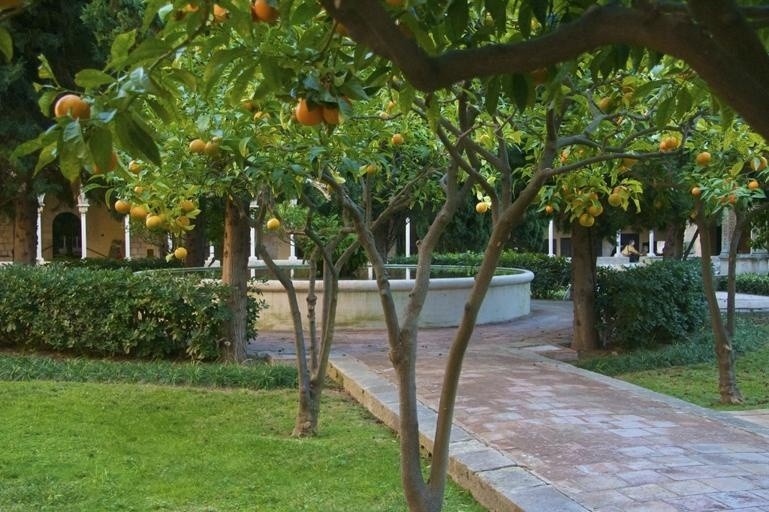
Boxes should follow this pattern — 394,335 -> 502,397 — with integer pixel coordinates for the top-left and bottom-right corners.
612,240 -> 625,256
621,239 -> 646,263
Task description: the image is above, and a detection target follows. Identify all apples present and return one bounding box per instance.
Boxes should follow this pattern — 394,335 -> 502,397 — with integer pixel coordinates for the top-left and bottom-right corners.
52,0 -> 769,260
